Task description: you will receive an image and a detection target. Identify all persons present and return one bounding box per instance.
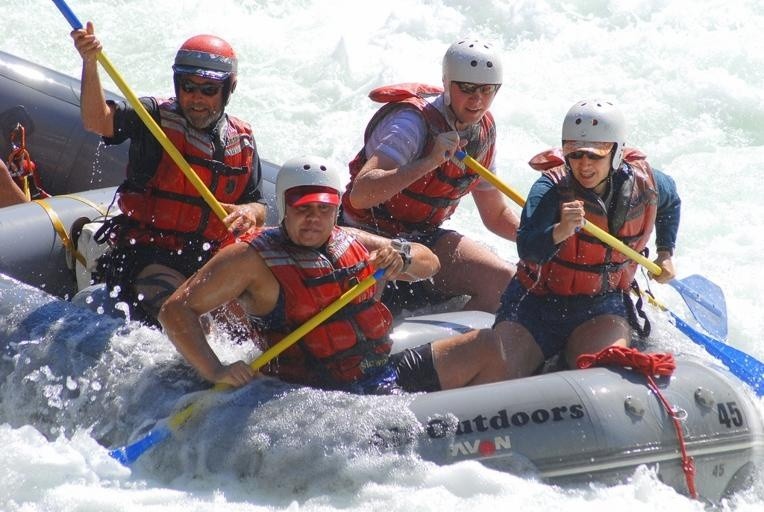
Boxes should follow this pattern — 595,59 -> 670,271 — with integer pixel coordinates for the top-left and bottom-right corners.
469,99 -> 682,386
157,155 -> 508,387
338,39 -> 513,313
0,159 -> 30,210
70,21 -> 268,336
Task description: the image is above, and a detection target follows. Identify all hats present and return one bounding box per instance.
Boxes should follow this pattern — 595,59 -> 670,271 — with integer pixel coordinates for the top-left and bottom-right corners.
561,141 -> 616,158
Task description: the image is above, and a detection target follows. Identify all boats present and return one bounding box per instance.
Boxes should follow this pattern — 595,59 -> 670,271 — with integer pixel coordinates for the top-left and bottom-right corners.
1,50 -> 764,507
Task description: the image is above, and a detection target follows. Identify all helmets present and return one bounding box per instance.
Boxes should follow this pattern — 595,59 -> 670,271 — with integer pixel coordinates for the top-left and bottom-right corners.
277,154 -> 341,223
442,39 -> 503,107
173,65 -> 229,81
286,186 -> 338,209
560,99 -> 623,170
173,34 -> 236,80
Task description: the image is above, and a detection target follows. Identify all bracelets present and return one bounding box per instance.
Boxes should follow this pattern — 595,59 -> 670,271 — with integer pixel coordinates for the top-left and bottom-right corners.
391,238 -> 411,273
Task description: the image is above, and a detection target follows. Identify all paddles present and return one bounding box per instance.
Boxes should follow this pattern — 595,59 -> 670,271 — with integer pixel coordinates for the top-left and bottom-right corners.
452,146 -> 764,400
580,214 -> 728,344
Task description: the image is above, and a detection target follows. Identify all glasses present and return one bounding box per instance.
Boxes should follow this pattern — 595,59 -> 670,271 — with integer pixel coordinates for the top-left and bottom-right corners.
571,152 -> 602,160
452,78 -> 498,96
178,76 -> 221,97
